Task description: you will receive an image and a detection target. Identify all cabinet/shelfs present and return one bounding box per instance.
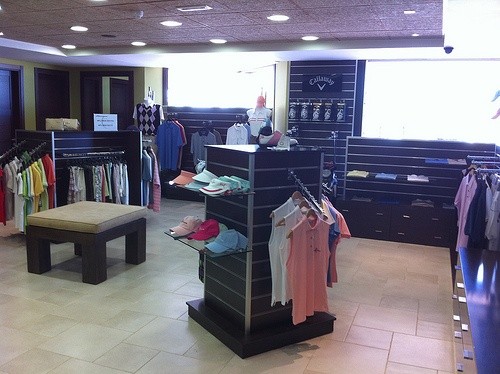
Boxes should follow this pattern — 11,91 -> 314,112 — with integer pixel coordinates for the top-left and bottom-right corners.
450,247 -> 500,374
336,196 -> 455,247
163,144 -> 336,358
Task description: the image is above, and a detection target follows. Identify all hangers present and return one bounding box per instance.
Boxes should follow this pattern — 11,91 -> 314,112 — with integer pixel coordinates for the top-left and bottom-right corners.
268,172 -> 331,239
142,140 -> 160,159
0,140 -> 127,183
166,112 -> 176,122
198,121 -> 215,133
235,115 -> 250,126
467,159 -> 500,191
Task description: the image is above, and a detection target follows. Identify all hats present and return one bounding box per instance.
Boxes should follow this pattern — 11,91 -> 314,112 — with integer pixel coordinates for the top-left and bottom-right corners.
204,229 -> 248,254
173,169 -> 218,192
187,219 -> 219,240
199,175 -> 250,196
169,216 -> 202,236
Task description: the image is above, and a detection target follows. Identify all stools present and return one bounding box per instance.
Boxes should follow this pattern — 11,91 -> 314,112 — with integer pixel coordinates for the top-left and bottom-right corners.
25,201 -> 148,284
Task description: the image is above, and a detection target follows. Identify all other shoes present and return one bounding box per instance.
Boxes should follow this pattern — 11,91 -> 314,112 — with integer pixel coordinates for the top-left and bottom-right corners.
259,131 -> 298,148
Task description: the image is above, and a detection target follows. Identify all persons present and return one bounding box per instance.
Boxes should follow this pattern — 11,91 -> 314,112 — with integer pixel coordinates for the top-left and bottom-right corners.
247,96 -> 272,144
